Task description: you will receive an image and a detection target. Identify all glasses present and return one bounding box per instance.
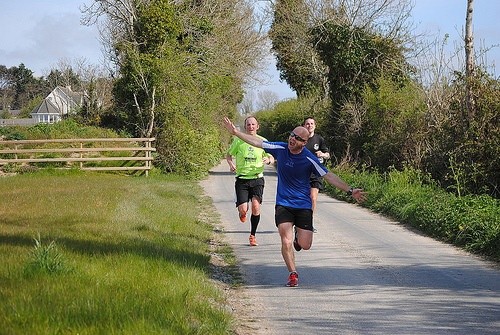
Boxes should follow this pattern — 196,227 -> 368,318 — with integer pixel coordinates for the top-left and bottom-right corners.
290,131 -> 305,142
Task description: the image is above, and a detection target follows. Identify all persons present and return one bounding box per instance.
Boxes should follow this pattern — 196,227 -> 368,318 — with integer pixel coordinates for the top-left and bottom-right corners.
304,117 -> 330,233
227,116 -> 275,245
225,116 -> 368,288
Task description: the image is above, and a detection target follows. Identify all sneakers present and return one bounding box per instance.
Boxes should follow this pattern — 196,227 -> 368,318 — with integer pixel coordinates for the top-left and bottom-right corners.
293,226 -> 302,251
239,211 -> 246,222
249,234 -> 258,246
286,272 -> 299,287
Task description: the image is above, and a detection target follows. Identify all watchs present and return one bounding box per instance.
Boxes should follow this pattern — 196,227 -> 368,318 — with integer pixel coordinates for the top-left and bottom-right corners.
348,188 -> 353,195
